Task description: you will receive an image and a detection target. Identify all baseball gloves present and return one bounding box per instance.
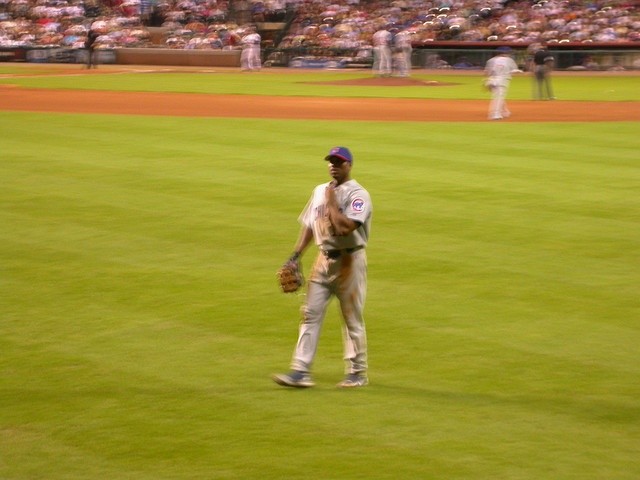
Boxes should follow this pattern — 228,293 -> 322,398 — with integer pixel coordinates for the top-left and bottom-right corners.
276,260 -> 303,293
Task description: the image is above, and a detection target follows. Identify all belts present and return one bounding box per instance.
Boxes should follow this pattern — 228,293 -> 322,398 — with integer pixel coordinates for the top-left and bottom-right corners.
323,246 -> 363,259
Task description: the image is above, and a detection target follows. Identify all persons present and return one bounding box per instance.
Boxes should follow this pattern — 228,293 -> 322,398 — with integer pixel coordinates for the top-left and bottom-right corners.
390,25 -> 412,76
241,27 -> 253,73
483,49 -> 522,121
83,24 -> 97,68
274,147 -> 372,392
374,24 -> 393,76
253,25 -> 262,71
531,43 -> 559,101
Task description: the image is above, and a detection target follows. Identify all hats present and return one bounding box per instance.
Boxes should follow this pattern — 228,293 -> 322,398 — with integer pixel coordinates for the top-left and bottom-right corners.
325,147 -> 352,161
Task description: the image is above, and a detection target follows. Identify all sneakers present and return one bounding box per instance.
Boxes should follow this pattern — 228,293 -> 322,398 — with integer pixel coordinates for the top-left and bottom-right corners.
272,369 -> 316,387
336,373 -> 368,387
